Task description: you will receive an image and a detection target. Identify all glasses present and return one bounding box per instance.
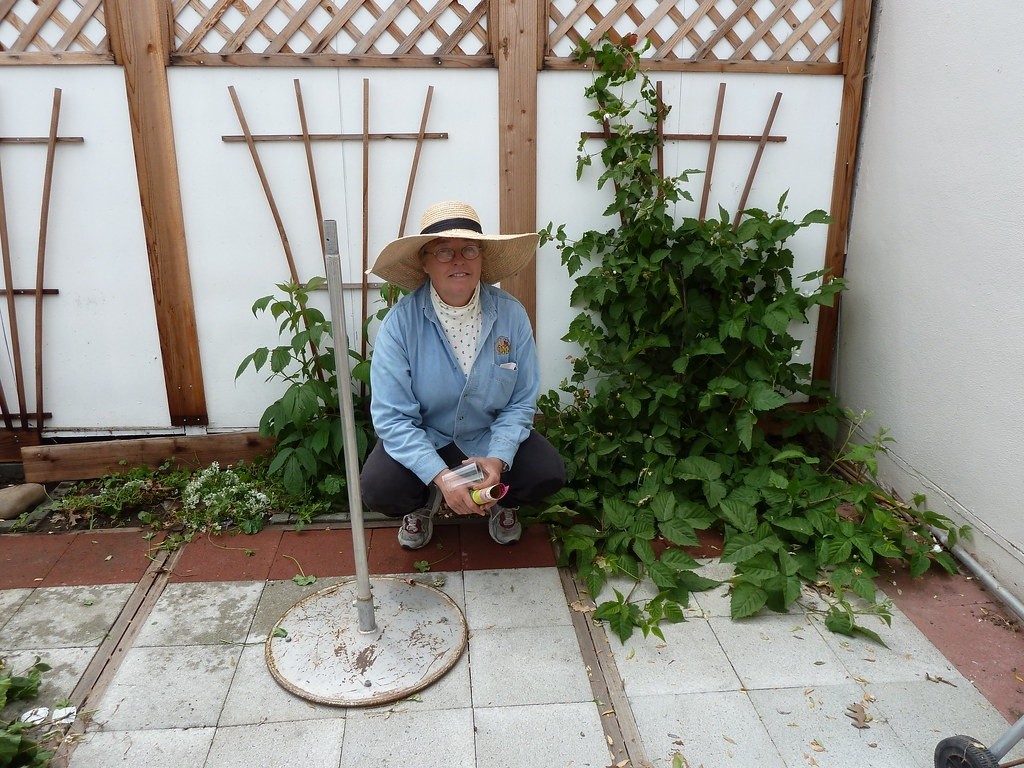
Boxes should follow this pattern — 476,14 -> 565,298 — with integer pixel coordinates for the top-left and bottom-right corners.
424,245 -> 482,263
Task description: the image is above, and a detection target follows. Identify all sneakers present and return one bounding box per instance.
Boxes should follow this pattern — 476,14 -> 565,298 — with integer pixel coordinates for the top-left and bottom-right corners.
488,504 -> 522,545
397,484 -> 442,549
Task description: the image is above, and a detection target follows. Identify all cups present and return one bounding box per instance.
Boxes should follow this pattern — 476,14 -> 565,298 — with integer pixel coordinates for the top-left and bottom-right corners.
441,461 -> 485,491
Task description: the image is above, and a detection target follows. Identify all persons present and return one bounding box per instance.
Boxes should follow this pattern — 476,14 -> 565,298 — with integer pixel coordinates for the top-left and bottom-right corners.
360,201 -> 566,550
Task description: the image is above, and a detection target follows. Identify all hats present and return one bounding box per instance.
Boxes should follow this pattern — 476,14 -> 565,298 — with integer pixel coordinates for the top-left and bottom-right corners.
371,201 -> 540,292
